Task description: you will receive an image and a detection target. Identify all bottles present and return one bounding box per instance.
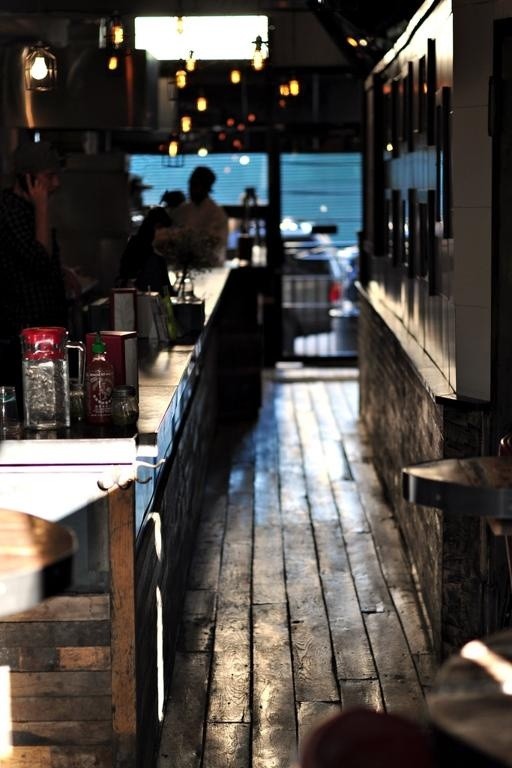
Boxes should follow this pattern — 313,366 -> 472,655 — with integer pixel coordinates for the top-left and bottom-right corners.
241,188 -> 258,233
111,384 -> 141,428
159,284 -> 178,340
85,329 -> 114,423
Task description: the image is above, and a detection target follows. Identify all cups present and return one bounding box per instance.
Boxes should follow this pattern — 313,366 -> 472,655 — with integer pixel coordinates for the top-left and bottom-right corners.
0,387 -> 21,436
20,325 -> 85,430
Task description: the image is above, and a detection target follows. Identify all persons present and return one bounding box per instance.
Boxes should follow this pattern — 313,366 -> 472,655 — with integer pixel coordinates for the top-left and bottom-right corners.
172,166 -> 227,257
0,138 -> 71,390
119,190 -> 185,290
127,178 -> 147,209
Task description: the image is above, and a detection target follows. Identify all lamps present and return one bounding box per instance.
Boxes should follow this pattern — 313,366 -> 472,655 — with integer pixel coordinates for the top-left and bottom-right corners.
23,41 -> 56,91
253,36 -> 262,68
